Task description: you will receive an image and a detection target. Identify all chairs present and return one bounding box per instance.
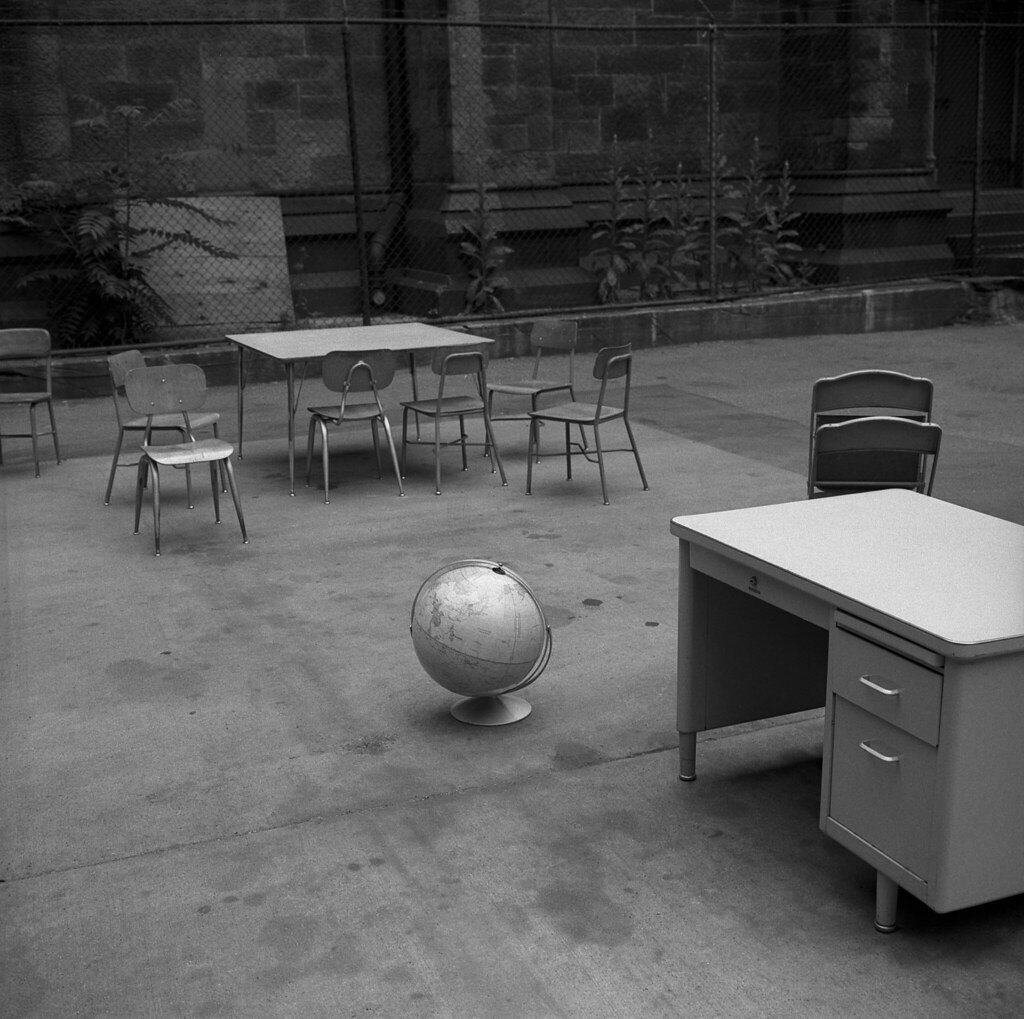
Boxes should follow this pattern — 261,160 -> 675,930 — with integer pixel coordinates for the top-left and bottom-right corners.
124,363 -> 250,556
525,342 -> 648,505
807,369 -> 945,500
306,349 -> 404,503
104,349 -> 228,511
0,326 -> 62,482
483,322 -> 590,464
399,343 -> 508,496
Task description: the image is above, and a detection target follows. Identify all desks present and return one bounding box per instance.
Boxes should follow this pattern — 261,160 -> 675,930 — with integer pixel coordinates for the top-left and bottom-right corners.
670,487 -> 1023,933
224,322 -> 496,498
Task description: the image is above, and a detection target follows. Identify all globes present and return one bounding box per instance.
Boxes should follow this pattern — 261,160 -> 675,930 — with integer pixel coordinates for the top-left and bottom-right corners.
410,557 -> 553,728
